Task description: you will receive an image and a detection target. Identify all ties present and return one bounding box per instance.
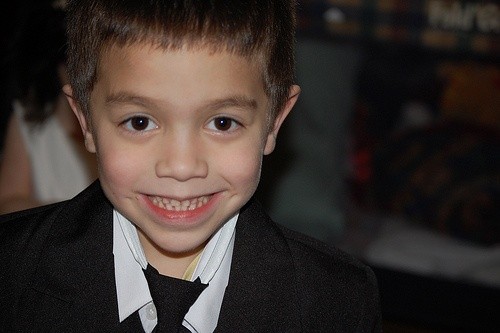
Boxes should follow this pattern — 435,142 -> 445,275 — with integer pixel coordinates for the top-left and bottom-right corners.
139,265 -> 212,333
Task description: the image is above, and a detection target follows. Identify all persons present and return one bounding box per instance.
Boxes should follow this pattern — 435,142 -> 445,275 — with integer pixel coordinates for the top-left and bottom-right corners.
1,0 -> 390,333
0,1 -> 101,216
291,1 -> 500,255
